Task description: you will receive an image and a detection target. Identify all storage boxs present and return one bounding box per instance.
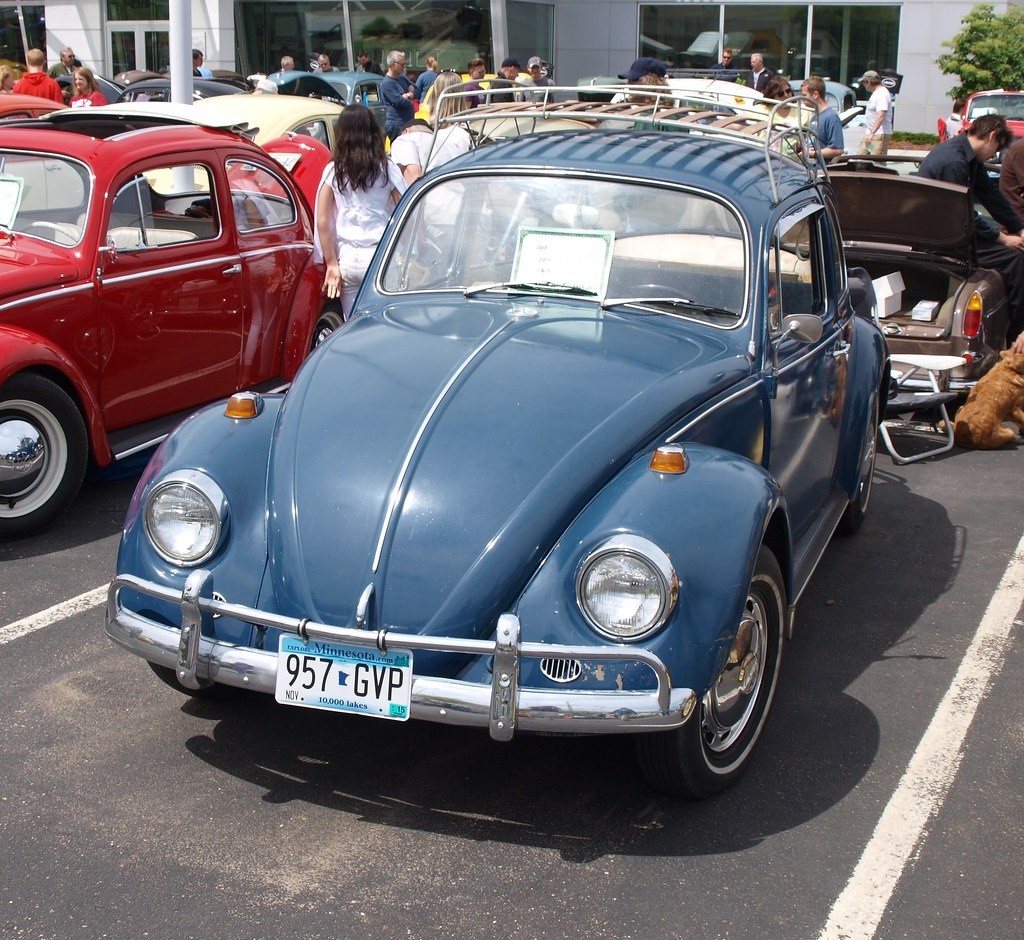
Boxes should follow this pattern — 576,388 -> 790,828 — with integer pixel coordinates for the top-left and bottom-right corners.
864,270 -> 939,323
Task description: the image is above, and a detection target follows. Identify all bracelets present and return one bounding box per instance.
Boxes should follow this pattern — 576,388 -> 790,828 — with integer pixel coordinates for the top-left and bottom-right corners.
408,94 -> 410,99
871,131 -> 875,133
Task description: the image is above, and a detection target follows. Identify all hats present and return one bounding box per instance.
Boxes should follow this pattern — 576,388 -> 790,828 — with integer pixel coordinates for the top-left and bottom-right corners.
618,56 -> 667,82
256,79 -> 278,96
857,70 -> 880,84
527,56 -> 542,68
389,118 -> 433,148
501,57 -> 525,72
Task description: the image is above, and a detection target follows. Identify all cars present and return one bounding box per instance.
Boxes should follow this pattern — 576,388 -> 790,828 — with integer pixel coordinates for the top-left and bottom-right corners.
959,88 -> 1024,141
105,70 -> 891,800
0,71 -> 386,539
816,155 -> 1011,464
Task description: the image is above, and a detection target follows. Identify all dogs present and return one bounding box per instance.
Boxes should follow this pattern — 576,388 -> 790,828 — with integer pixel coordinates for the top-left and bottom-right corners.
940,342 -> 1024,451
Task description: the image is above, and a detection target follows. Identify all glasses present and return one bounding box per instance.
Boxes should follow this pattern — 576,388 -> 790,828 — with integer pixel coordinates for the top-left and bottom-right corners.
393,61 -> 406,68
777,88 -> 792,97
320,63 -> 328,67
990,130 -> 1005,152
723,56 -> 732,59
63,55 -> 76,58
474,69 -> 486,75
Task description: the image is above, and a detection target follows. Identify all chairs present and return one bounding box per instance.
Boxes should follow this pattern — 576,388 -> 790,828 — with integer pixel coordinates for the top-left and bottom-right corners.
878,354 -> 975,464
99,226 -> 197,250
17,221 -> 84,248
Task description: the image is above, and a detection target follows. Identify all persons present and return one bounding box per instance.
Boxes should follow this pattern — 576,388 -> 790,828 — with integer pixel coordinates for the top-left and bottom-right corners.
193,49 -> 203,77
912,98 -> 1024,354
0,47 -> 108,108
708,49 -> 894,171
611,58 -> 690,234
311,106 -> 408,322
267,49 -> 555,289
